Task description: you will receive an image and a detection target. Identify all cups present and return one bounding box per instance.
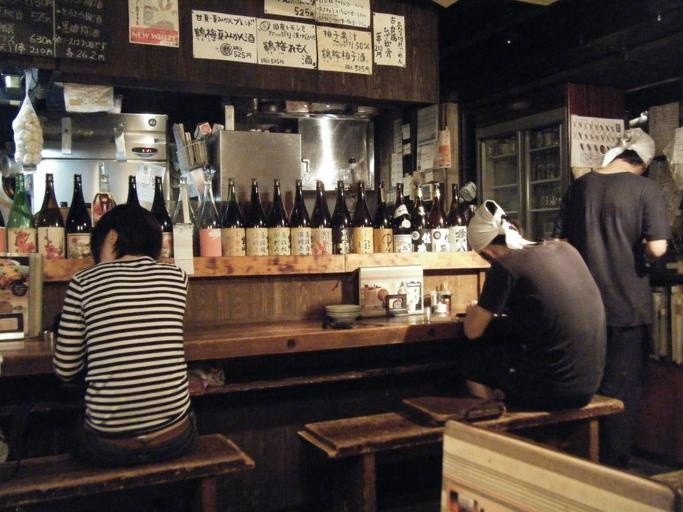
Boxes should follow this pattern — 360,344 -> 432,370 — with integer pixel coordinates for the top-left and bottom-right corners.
432,303 -> 447,316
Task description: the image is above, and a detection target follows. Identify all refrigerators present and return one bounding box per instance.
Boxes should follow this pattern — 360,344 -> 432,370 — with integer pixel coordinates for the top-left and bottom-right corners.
475,82 -> 626,240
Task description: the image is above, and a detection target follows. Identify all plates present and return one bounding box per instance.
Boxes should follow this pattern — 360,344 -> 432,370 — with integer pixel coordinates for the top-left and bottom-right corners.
326,304 -> 360,323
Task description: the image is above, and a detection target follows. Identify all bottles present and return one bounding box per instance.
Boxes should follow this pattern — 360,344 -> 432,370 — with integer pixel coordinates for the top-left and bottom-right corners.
371,182 -> 394,254
265,178 -> 292,255
222,177 -> 246,257
35,173 -> 66,258
391,182 -> 414,253
194,164 -> 224,258
431,287 -> 451,311
331,180 -> 353,255
351,180 -> 374,255
170,175 -> 198,259
310,181 -> 332,256
288,178 -> 312,256
149,176 -> 173,259
244,179 -> 269,256
427,183 -> 448,252
64,173 -> 92,258
6,172 -> 37,253
446,183 -> 466,253
125,175 -> 141,206
90,172 -> 117,226
411,185 -> 432,253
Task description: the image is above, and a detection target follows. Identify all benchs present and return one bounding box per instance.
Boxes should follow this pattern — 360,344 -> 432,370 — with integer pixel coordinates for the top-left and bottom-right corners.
297,396 -> 624,512
0,433 -> 256,512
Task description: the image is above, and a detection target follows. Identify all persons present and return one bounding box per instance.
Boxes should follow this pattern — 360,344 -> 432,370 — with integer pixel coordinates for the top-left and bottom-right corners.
552,128 -> 674,468
454,198 -> 609,410
50,202 -> 199,466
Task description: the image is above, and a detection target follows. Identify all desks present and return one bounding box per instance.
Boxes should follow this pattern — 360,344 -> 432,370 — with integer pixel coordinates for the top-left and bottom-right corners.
0,306 -> 507,417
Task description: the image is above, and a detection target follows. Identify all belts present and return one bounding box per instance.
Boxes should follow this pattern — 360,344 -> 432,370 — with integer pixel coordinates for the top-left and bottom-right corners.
97,418 -> 191,448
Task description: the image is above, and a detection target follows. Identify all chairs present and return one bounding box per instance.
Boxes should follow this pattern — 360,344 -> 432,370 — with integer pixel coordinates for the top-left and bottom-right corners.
441,419 -> 681,512
